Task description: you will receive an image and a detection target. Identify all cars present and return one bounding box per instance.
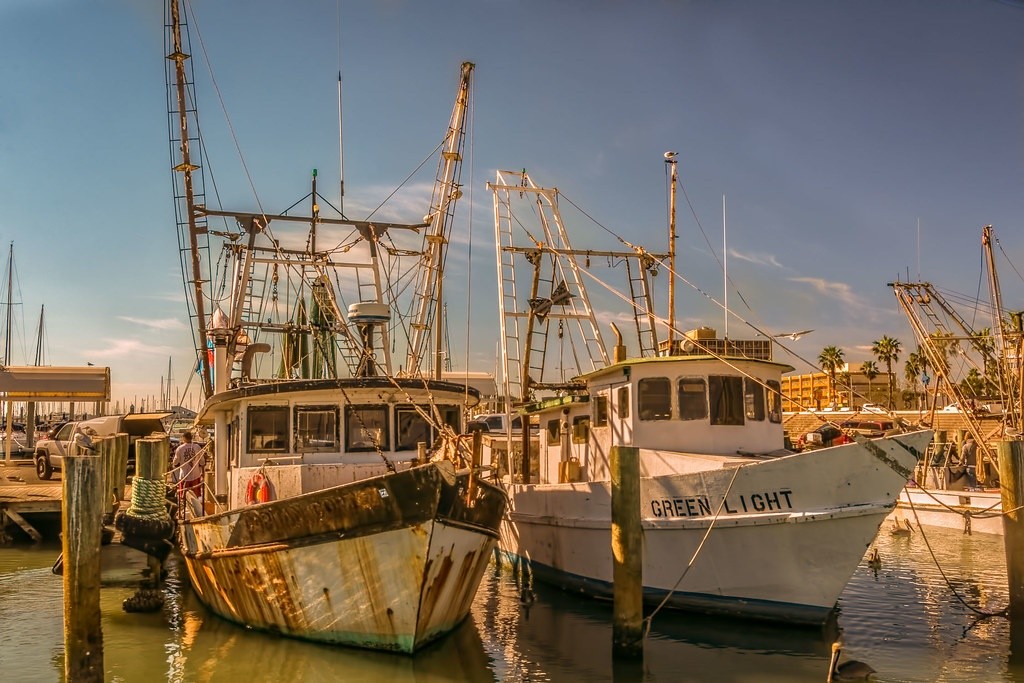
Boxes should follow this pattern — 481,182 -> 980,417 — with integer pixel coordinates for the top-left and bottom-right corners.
939,400 -> 992,414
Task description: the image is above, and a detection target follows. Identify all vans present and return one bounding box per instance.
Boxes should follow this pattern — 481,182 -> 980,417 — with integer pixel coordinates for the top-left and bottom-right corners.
862,403 -> 885,412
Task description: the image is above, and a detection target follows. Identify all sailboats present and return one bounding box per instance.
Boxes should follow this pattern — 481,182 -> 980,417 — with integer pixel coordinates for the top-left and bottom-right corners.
0,238 -> 214,468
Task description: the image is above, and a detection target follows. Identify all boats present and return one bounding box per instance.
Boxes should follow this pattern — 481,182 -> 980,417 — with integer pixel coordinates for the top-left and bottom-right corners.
159,0 -> 508,657
484,149 -> 936,629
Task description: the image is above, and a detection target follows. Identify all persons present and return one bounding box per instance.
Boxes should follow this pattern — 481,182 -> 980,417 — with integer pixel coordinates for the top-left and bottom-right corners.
956,432 -> 978,480
173,432 -> 205,499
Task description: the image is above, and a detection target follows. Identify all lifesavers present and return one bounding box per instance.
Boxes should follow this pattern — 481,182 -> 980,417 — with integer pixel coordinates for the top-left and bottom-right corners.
245,475 -> 271,505
185,490 -> 202,519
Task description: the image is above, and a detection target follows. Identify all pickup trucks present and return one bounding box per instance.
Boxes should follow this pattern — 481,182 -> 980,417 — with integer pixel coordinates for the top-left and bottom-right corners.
32,421 -> 136,485
